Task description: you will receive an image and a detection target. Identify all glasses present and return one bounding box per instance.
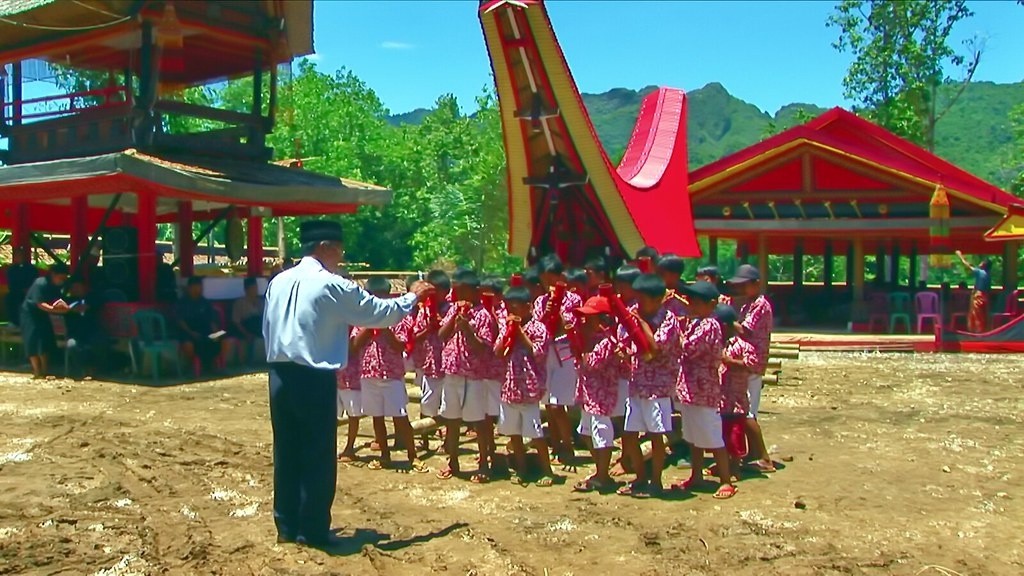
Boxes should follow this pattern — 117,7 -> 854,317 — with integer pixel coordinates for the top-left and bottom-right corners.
336,247 -> 346,258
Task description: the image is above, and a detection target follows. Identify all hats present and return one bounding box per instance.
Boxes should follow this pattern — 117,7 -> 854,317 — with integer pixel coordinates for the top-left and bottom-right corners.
244,276 -> 257,287
301,221 -> 344,245
571,295 -> 610,316
726,264 -> 759,285
678,281 -> 720,299
187,275 -> 207,284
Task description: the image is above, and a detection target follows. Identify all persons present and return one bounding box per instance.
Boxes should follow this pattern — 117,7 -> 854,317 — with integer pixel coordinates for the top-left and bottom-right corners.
898,281 -> 969,323
0,246 -> 233,380
232,275 -> 264,362
262,220 -> 435,548
336,246 -> 777,498
954,250 -> 992,333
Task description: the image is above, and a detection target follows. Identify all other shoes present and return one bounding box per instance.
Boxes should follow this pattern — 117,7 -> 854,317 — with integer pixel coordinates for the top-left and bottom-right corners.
549,449 -> 575,465
573,476 -> 615,491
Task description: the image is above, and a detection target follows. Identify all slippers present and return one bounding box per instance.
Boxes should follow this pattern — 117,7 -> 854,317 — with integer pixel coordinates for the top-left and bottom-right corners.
414,441 -> 429,452
712,486 -> 739,498
534,471 -> 553,487
434,445 -> 450,456
411,459 -> 430,472
470,466 -> 490,483
368,457 -> 391,470
510,472 -> 530,484
741,460 -> 777,473
677,478 -> 707,491
617,480 -> 663,498
436,466 -> 459,479
702,466 -> 719,476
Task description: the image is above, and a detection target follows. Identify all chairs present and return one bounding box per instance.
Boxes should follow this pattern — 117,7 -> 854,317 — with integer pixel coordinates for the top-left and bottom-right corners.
991,292 -> 1012,331
128,309 -> 180,381
192,304 -> 230,374
948,289 -> 971,331
865,291 -> 888,332
913,291 -> 941,334
887,291 -> 912,335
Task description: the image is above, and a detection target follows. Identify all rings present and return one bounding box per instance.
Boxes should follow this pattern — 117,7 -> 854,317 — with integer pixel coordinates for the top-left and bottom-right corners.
424,282 -> 428,286
